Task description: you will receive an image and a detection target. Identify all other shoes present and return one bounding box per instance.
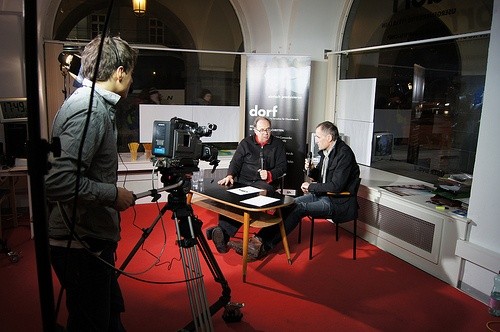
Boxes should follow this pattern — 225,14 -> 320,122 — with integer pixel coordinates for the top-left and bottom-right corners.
227,237 -> 264,256
206,226 -> 218,240
235,246 -> 267,262
212,227 -> 227,253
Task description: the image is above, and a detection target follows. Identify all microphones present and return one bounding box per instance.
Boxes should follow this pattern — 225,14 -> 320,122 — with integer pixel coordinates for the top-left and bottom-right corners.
306,152 -> 312,176
260,152 -> 264,171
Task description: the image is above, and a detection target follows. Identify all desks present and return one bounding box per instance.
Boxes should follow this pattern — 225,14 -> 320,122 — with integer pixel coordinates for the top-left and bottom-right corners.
186,179 -> 296,283
0,166 -> 34,239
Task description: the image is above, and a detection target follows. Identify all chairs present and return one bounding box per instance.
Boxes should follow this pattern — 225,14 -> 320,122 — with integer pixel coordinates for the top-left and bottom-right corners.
299,177 -> 361,260
265,173 -> 286,215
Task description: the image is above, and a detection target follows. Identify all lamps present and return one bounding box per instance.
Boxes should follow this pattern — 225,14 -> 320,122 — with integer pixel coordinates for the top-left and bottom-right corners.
129,0 -> 150,21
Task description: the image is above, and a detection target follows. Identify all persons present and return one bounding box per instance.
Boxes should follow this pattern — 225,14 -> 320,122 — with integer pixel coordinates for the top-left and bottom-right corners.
228,122 -> 362,261
202,117 -> 287,254
117,86 -> 213,152
46,35 -> 134,332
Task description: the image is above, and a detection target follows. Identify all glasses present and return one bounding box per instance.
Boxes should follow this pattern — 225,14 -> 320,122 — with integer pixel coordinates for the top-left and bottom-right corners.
254,128 -> 273,134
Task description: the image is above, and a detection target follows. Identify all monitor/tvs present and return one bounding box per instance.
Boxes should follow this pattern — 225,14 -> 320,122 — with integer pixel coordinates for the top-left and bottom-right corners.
371,132 -> 394,161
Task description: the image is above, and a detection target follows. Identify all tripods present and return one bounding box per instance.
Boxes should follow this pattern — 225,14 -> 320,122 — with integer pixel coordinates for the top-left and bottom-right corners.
115,158 -> 246,332
